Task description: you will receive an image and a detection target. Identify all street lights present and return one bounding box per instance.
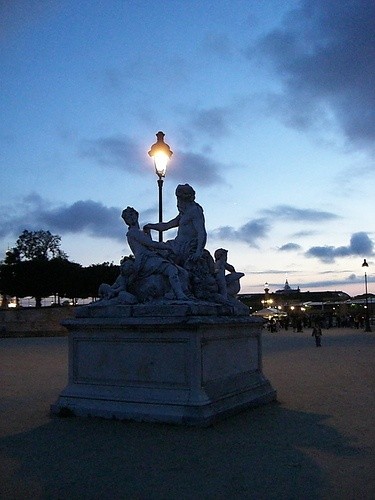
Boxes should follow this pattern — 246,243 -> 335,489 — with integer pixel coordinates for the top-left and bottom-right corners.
362,259 -> 374,332
148,130 -> 173,244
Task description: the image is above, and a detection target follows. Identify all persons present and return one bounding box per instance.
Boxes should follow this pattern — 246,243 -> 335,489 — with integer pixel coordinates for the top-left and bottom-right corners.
270,311 -> 366,333
311,322 -> 322,347
99,183 -> 240,304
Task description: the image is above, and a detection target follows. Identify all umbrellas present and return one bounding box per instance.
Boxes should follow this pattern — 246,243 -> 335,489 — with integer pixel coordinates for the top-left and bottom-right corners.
251,307 -> 287,320
